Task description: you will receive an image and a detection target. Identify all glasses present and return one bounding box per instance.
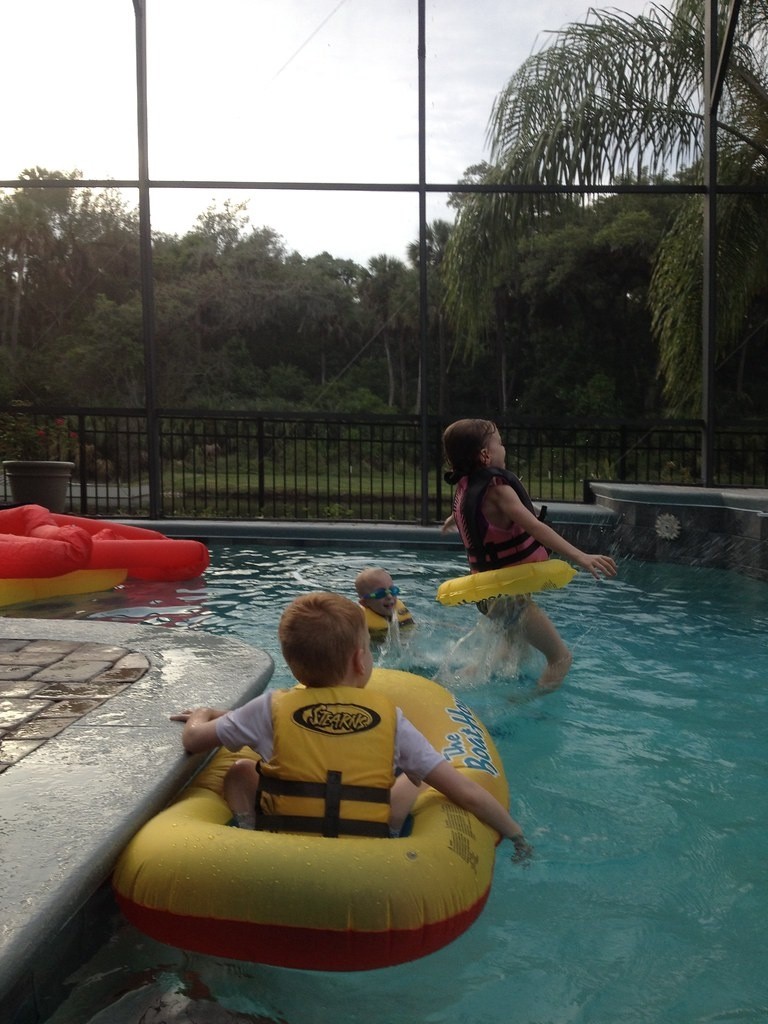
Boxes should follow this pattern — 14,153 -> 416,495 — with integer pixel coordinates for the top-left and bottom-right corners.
359,586 -> 399,599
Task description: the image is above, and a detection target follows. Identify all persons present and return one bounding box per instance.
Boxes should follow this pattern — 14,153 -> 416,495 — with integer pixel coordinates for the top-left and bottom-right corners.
166,587 -> 535,865
440,418 -> 617,705
356,566 -> 399,617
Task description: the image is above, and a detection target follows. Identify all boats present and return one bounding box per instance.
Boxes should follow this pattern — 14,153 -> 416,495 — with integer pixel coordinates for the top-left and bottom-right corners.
0,506 -> 210,610
110,670 -> 512,977
